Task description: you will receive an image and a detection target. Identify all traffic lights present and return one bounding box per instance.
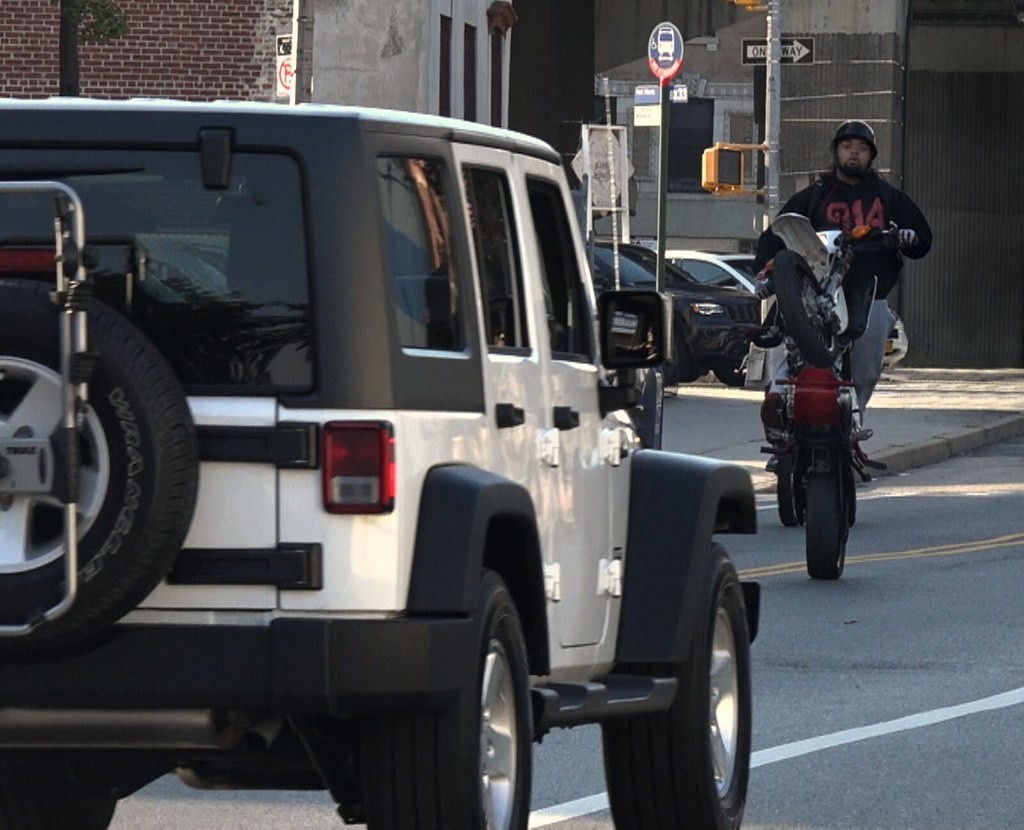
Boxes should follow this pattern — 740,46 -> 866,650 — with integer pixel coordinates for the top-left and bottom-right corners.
698,143 -> 746,192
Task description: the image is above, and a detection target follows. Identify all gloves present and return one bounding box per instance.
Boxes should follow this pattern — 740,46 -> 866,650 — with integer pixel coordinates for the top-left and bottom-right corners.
754,268 -> 776,299
900,226 -> 921,249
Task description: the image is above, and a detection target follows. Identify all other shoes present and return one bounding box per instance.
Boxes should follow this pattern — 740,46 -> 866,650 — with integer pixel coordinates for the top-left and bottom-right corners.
759,393 -> 786,443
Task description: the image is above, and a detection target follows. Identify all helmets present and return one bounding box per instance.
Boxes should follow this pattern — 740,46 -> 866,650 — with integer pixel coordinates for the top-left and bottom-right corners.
831,118 -> 878,159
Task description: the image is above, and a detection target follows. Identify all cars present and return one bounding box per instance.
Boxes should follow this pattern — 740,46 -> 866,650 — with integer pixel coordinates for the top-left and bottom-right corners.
583,240 -> 762,388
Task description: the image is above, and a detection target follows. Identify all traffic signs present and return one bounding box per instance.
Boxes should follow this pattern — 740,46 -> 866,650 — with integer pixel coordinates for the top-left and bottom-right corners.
739,35 -> 818,69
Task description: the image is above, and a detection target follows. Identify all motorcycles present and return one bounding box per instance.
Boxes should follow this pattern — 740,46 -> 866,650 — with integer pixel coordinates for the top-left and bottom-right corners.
759,210 -> 906,581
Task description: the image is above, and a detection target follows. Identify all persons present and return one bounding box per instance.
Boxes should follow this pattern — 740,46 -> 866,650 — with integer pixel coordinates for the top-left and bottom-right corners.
752,119 -> 934,444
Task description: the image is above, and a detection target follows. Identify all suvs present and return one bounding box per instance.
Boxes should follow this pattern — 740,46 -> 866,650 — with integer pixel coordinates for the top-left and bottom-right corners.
0,91 -> 764,830
